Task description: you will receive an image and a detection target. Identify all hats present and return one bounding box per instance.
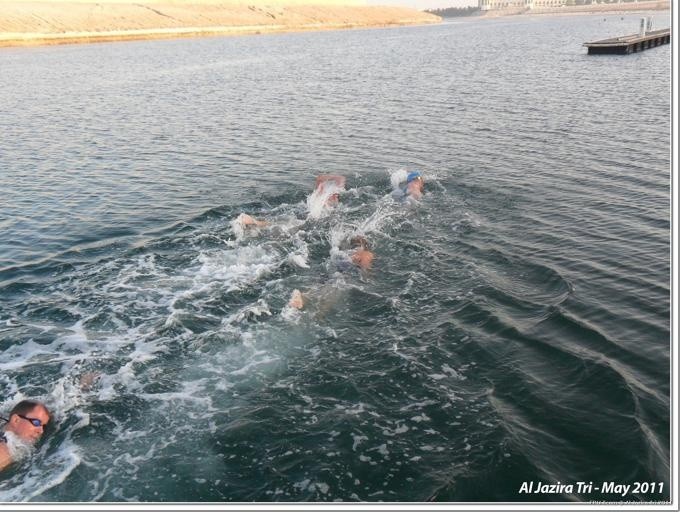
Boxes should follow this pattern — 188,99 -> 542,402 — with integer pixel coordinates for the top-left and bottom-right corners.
406,171 -> 422,183
18,414 -> 46,429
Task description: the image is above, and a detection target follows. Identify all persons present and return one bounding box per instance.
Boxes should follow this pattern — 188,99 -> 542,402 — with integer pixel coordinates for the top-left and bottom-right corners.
237,175 -> 345,226
290,236 -> 373,309
0,399 -> 50,470
400,172 -> 424,202
